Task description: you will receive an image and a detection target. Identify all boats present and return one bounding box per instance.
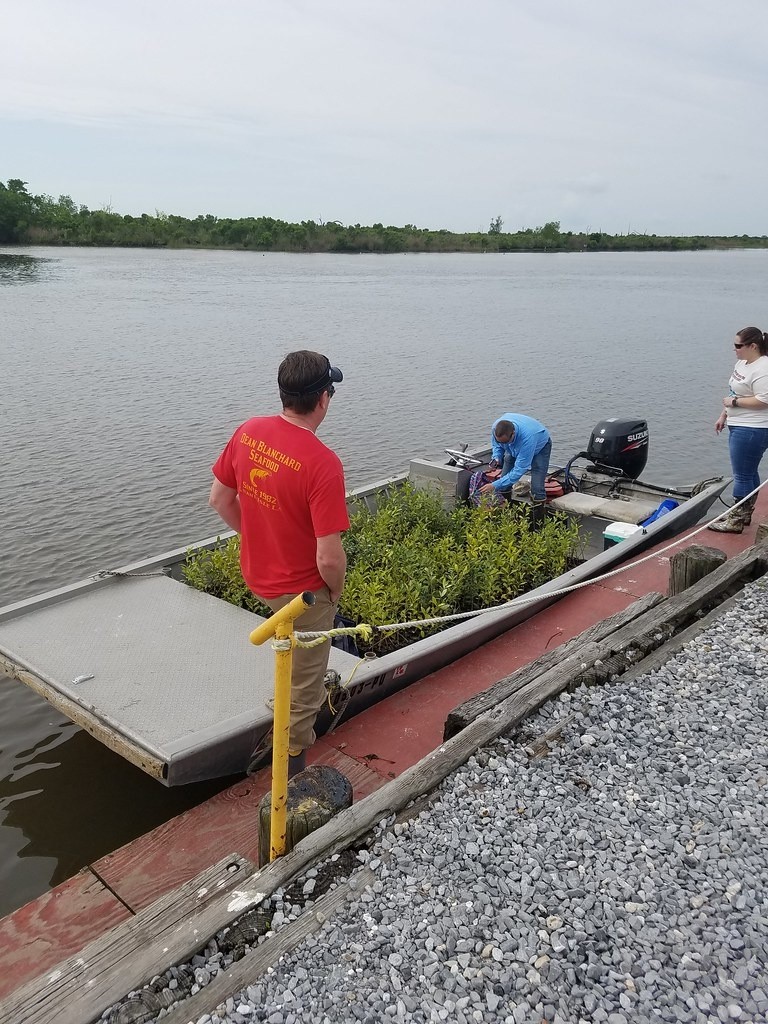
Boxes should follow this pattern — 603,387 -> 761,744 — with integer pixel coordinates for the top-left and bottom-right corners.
0,420 -> 732,789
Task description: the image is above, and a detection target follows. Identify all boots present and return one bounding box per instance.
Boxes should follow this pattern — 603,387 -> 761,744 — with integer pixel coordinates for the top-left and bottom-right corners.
500,490 -> 511,503
528,498 -> 546,532
720,491 -> 759,526
710,497 -> 751,533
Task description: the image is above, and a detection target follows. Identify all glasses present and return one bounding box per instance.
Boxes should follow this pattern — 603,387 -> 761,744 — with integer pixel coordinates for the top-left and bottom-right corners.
494,433 -> 513,444
734,343 -> 751,349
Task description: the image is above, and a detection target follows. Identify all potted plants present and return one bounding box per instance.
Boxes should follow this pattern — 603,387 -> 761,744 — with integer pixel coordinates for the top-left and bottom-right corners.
184,473 -> 595,659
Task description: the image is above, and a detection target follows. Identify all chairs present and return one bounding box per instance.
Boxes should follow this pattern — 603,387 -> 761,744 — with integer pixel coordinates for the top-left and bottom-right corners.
469,457 -> 502,480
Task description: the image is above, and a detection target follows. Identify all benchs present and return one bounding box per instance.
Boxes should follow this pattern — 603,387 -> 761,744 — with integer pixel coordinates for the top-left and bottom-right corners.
511,486 -> 659,525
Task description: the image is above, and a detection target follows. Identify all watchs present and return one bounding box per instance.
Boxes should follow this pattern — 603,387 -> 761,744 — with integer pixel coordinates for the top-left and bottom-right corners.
732,397 -> 739,407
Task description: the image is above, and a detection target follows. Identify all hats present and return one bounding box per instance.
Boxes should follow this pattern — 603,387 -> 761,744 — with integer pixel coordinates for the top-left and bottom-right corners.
279,354 -> 343,398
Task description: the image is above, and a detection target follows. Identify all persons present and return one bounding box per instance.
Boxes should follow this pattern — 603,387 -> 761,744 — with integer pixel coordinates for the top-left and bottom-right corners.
207,350 -> 351,776
708,326 -> 768,534
478,413 -> 552,500
